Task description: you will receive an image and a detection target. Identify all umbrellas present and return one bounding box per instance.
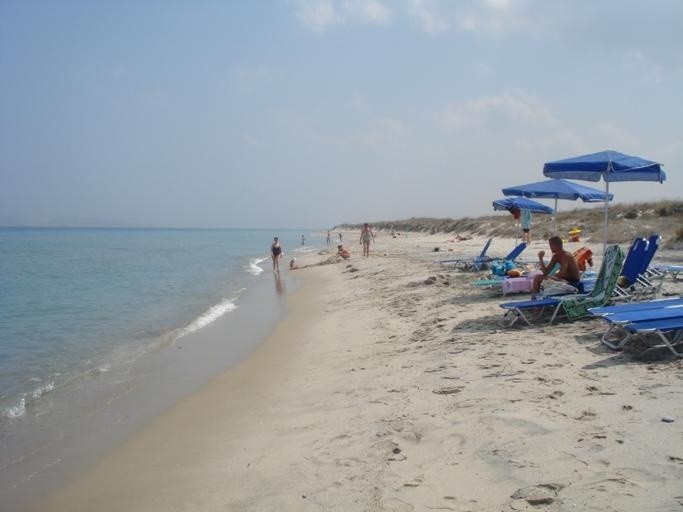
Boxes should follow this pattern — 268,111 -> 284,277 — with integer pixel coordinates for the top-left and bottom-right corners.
542,149 -> 666,257
491,196 -> 554,248
501,178 -> 614,237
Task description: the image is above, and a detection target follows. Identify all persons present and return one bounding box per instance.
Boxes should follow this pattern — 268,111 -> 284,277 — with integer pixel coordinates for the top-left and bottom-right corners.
270,236 -> 284,271
326,230 -> 332,248
520,208 -> 533,248
360,223 -> 375,259
333,243 -> 351,261
288,257 -> 300,270
527,235 -> 580,321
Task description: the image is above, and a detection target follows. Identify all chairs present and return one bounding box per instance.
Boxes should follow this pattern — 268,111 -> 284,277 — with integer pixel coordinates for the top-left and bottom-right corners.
432,233 -> 682,364
